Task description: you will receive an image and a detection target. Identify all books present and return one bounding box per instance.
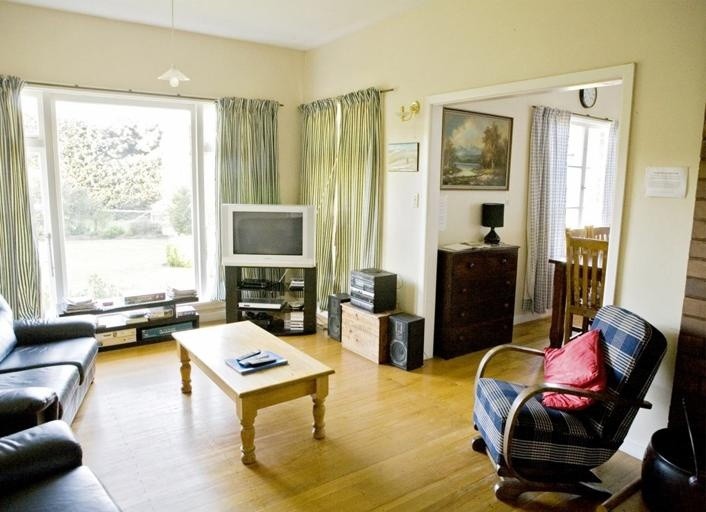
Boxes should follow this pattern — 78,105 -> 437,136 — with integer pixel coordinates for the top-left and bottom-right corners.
60,287 -> 200,328
281,308 -> 304,331
225,348 -> 288,375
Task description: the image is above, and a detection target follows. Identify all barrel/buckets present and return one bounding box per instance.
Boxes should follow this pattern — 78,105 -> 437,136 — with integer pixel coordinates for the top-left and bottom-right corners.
641,399 -> 706,512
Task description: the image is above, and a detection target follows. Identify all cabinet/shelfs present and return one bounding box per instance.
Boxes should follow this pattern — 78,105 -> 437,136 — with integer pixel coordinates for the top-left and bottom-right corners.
54,293 -> 200,353
430,239 -> 520,364
336,299 -> 407,367
220,265 -> 319,336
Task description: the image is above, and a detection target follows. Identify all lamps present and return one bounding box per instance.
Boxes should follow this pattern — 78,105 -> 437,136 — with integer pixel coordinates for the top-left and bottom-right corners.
477,201 -> 506,246
156,1 -> 191,90
397,99 -> 422,122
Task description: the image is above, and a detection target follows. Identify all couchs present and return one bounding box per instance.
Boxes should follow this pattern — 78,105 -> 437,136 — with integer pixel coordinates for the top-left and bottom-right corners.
0,293 -> 101,436
0,419 -> 121,512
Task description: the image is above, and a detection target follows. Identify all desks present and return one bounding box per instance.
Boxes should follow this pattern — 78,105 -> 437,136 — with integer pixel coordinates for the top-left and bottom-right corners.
547,250 -> 607,347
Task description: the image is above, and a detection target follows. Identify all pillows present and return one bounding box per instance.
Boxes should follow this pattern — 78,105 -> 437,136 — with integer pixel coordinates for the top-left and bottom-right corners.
538,325 -> 610,413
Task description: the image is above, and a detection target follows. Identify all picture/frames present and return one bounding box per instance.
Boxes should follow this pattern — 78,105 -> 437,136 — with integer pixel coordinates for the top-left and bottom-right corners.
437,103 -> 516,193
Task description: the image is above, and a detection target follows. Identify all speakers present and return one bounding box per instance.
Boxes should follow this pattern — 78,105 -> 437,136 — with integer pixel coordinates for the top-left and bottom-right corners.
326,293 -> 350,342
387,312 -> 424,372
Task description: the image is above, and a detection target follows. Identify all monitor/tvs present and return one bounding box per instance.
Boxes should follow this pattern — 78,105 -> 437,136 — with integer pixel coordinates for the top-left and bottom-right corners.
220,204 -> 317,268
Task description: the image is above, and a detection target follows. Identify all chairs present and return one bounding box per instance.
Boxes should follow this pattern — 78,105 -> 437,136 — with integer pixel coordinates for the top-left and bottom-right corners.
588,222 -> 609,257
558,234 -> 611,348
561,224 -> 591,262
467,303 -> 670,506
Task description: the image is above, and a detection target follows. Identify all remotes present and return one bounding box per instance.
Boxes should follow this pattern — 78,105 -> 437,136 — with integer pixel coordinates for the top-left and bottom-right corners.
239,353 -> 269,367
248,357 -> 276,366
236,348 -> 260,362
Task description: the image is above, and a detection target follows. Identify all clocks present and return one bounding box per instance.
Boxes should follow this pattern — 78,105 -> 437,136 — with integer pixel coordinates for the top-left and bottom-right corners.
578,84 -> 597,110
